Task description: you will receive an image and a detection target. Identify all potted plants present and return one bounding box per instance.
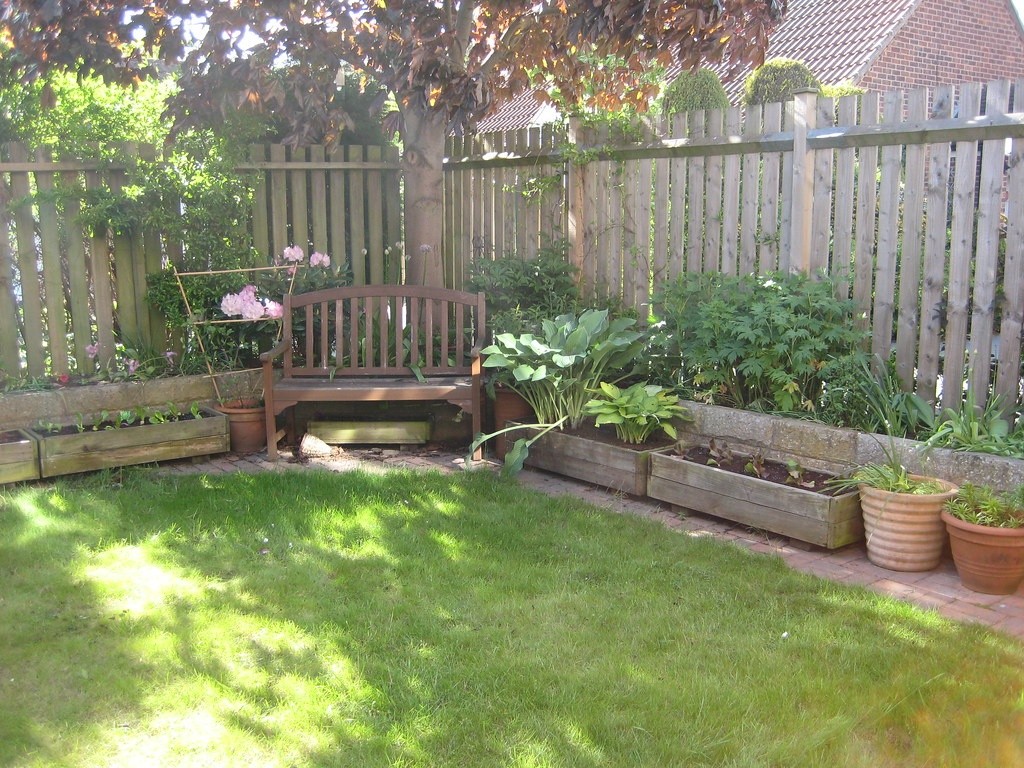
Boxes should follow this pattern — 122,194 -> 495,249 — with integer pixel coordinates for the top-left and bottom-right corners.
819,348 -> 956,573
939,485 -> 1024,593
468,309 -> 690,497
218,397 -> 269,455
647,441 -> 864,550
26,403 -> 231,477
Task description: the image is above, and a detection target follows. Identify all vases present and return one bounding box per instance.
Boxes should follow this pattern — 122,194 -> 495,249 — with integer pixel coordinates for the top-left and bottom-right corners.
0,427 -> 40,487
485,308 -> 546,459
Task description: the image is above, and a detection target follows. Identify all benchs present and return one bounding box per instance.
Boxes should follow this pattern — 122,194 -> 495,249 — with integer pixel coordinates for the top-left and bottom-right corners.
259,285 -> 485,462
